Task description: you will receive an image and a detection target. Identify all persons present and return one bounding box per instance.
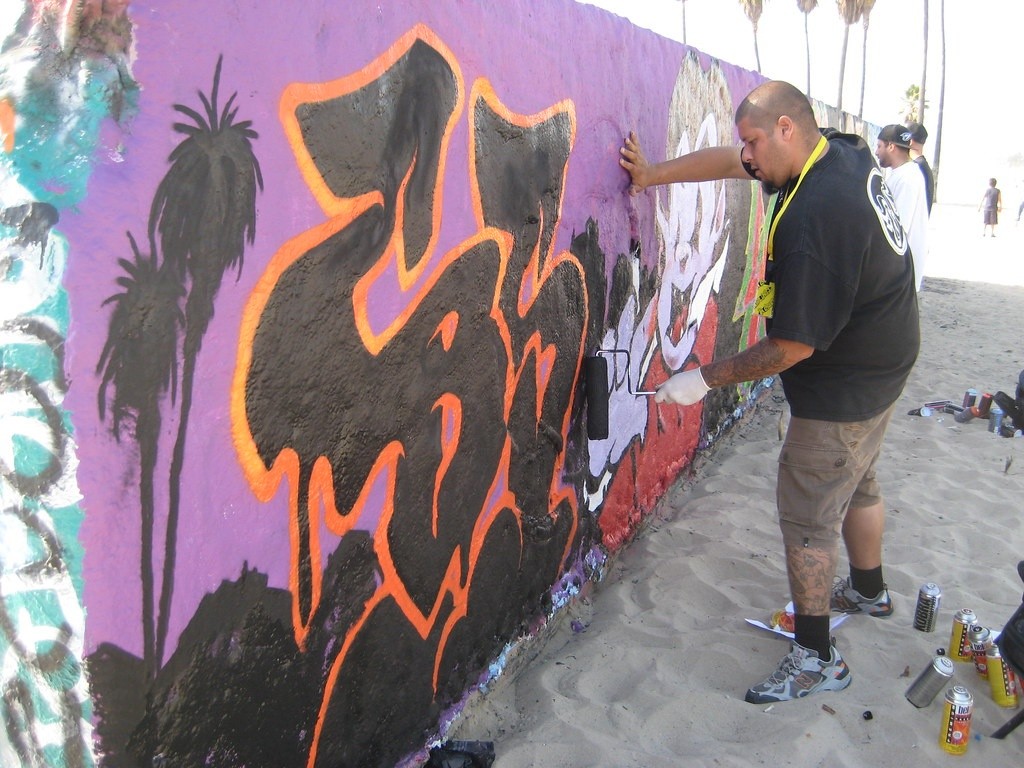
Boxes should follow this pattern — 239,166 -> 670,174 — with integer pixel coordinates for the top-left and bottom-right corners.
977,178 -> 1002,238
618,81 -> 920,704
875,124 -> 930,293
907,124 -> 935,215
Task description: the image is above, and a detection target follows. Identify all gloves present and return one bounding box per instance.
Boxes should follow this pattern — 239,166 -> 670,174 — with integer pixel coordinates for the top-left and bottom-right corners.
654,366 -> 713,406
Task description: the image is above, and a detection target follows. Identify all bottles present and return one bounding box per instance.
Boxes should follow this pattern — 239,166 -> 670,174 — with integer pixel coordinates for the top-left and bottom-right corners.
924,400 -> 953,411
913,583 -> 941,632
962,388 -> 978,407
970,626 -> 994,678
977,393 -> 994,416
905,655 -> 954,708
1000,421 -> 1023,438
987,408 -> 1005,433
940,685 -> 975,755
985,640 -> 1018,710
944,403 -> 964,415
955,406 -> 980,422
907,407 -> 931,417
949,608 -> 979,663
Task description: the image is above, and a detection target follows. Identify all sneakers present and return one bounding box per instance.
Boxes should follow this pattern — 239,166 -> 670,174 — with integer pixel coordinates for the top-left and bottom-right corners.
830,576 -> 894,617
745,640 -> 853,703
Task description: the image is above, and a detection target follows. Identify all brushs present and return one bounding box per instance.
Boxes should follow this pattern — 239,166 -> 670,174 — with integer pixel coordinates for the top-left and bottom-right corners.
584,348 -> 660,441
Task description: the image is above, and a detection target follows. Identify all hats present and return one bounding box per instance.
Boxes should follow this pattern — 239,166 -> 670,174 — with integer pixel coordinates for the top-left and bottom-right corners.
908,123 -> 929,143
878,124 -> 918,150
